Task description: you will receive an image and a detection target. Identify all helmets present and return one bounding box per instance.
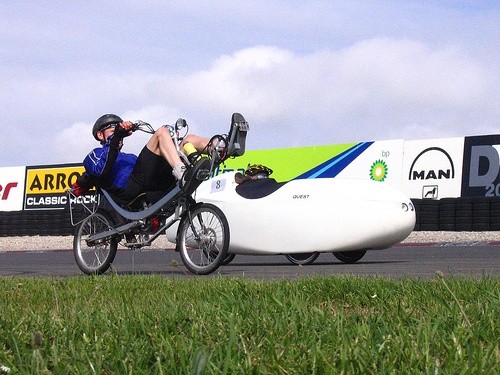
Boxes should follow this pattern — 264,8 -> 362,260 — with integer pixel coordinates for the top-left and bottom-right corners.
92,114 -> 123,141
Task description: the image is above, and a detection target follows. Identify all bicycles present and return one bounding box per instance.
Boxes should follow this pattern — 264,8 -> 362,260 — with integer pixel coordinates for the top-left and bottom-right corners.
66,118 -> 231,275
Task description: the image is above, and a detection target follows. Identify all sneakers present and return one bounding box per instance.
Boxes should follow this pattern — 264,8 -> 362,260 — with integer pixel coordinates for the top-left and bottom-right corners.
179,157 -> 211,195
227,113 -> 247,156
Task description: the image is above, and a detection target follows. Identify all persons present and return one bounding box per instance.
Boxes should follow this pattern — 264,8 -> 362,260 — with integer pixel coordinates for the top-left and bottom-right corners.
235,164 -> 273,184
84,111 -> 251,206
